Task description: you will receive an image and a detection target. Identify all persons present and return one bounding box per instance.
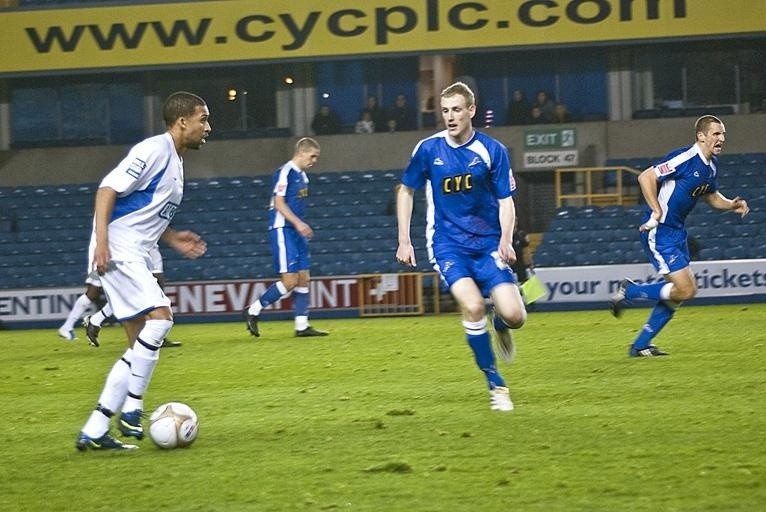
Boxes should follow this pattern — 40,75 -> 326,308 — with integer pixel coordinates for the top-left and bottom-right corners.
509,215 -> 536,313
504,88 -> 577,125
312,92 -> 436,136
242,137 -> 329,338
608,115 -> 750,356
395,83 -> 528,411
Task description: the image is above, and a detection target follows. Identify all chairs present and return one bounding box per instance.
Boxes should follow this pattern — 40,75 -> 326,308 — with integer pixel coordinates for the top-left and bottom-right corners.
0,104 -> 450,290
532,153 -> 766,269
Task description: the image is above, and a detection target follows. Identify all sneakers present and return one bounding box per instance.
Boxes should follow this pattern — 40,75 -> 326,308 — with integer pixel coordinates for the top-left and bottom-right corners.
243,306 -> 260,336
609,278 -> 633,317
57,325 -> 72,341
83,315 -> 99,347
119,409 -> 145,439
488,385 -> 512,410
76,429 -> 138,449
630,345 -> 668,356
492,320 -> 515,364
161,338 -> 180,347
295,327 -> 327,336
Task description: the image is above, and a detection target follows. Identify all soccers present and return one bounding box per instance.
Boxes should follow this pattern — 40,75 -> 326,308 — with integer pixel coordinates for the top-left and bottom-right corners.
150,402 -> 198,449
